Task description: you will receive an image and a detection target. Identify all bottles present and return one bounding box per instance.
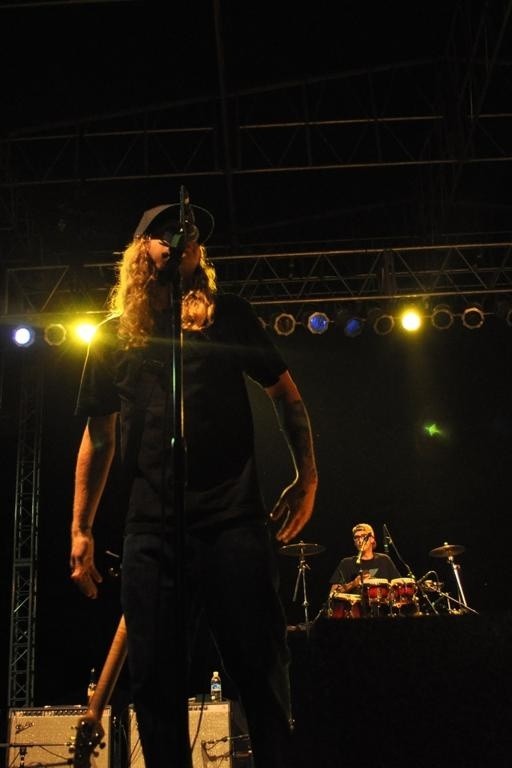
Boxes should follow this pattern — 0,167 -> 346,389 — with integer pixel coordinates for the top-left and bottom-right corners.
87,668 -> 100,700
209,671 -> 222,701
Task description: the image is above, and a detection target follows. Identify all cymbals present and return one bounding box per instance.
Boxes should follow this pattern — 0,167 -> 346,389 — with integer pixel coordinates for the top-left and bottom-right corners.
276,543 -> 323,555
433,546 -> 466,556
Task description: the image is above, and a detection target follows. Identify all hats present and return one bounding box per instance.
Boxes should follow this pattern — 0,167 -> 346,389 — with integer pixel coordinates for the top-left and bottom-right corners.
134,203 -> 215,245
352,523 -> 375,537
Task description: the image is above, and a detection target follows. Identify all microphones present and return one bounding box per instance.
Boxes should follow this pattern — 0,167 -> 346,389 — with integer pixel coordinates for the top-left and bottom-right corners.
183,193 -> 200,243
382,527 -> 390,555
355,534 -> 370,563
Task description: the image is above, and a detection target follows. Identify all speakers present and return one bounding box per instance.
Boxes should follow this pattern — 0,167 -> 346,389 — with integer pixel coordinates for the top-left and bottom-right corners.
5,706 -> 112,767
128,701 -> 232,767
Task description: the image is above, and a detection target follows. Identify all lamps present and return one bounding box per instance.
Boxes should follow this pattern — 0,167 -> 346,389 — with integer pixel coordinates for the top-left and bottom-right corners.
0,300 -> 511,349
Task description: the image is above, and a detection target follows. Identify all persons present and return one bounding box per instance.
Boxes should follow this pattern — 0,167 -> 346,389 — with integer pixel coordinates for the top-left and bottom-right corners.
65,200 -> 321,765
327,518 -> 407,610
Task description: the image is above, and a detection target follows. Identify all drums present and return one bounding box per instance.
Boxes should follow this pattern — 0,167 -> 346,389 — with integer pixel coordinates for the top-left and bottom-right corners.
329,592 -> 362,619
362,578 -> 391,612
389,579 -> 417,608
